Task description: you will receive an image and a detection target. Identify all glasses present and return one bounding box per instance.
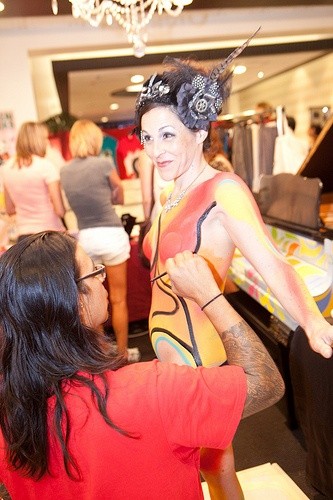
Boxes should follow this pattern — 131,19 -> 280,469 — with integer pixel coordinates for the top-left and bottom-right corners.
75,264 -> 107,284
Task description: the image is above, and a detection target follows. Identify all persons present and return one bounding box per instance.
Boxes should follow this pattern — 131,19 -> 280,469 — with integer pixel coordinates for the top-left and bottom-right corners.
0,122 -> 69,244
135,56 -> 333,500
201,126 -> 234,173
130,124 -> 160,270
1,231 -> 287,500
59,119 -> 131,370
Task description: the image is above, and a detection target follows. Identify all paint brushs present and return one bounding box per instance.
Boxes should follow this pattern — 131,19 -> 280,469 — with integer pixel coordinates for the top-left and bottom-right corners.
149,271 -> 169,283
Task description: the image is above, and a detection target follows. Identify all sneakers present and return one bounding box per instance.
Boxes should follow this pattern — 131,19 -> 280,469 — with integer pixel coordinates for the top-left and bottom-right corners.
127,347 -> 140,363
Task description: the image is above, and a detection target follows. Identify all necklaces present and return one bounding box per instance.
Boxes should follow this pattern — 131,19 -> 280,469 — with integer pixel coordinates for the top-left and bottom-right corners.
161,164 -> 209,213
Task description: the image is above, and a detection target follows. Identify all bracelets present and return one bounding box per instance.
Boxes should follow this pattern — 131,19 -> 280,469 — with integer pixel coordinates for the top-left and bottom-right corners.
197,292 -> 223,309
